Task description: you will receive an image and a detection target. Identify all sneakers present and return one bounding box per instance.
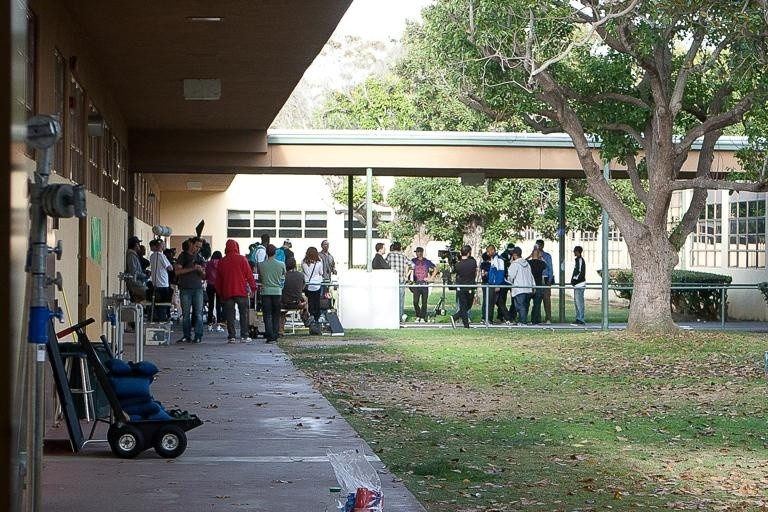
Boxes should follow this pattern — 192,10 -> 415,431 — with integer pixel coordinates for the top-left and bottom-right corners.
227,338 -> 235,344
176,336 -> 191,343
450,315 -> 456,330
192,337 -> 201,343
207,325 -> 213,332
419,318 -> 425,324
304,316 -> 313,327
239,337 -> 252,343
216,326 -> 224,333
414,317 -> 419,323
480,314 -> 584,327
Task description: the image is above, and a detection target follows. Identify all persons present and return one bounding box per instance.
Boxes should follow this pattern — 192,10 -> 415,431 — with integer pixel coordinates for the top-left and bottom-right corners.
124,233 -> 337,345
408,246 -> 439,324
372,241 -> 417,323
450,239 -> 554,329
569,244 -> 586,327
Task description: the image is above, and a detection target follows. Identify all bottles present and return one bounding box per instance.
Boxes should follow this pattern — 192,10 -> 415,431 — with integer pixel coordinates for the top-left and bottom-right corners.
323,487 -> 347,512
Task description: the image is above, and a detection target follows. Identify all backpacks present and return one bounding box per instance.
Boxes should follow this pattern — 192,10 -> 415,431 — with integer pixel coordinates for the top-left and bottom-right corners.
248,245 -> 264,267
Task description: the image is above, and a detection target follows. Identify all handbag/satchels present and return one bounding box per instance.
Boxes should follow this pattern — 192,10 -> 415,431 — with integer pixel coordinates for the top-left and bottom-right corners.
304,285 -> 308,297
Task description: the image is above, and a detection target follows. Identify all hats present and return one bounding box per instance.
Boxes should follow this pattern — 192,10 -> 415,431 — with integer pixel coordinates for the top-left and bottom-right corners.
413,247 -> 422,252
128,236 -> 141,244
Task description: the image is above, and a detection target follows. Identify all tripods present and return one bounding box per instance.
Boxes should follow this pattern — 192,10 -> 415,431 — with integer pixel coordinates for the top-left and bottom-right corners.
426,280 -> 457,321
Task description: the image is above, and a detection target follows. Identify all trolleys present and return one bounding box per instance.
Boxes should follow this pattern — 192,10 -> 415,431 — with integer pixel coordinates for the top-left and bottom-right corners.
57,317 -> 204,461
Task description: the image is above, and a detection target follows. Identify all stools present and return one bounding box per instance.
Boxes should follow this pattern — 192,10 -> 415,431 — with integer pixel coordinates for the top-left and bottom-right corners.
53,352 -> 96,423
280,308 -> 300,336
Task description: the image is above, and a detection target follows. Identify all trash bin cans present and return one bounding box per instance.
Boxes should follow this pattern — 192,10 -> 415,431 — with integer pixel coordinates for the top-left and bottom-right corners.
57,341 -> 118,419
